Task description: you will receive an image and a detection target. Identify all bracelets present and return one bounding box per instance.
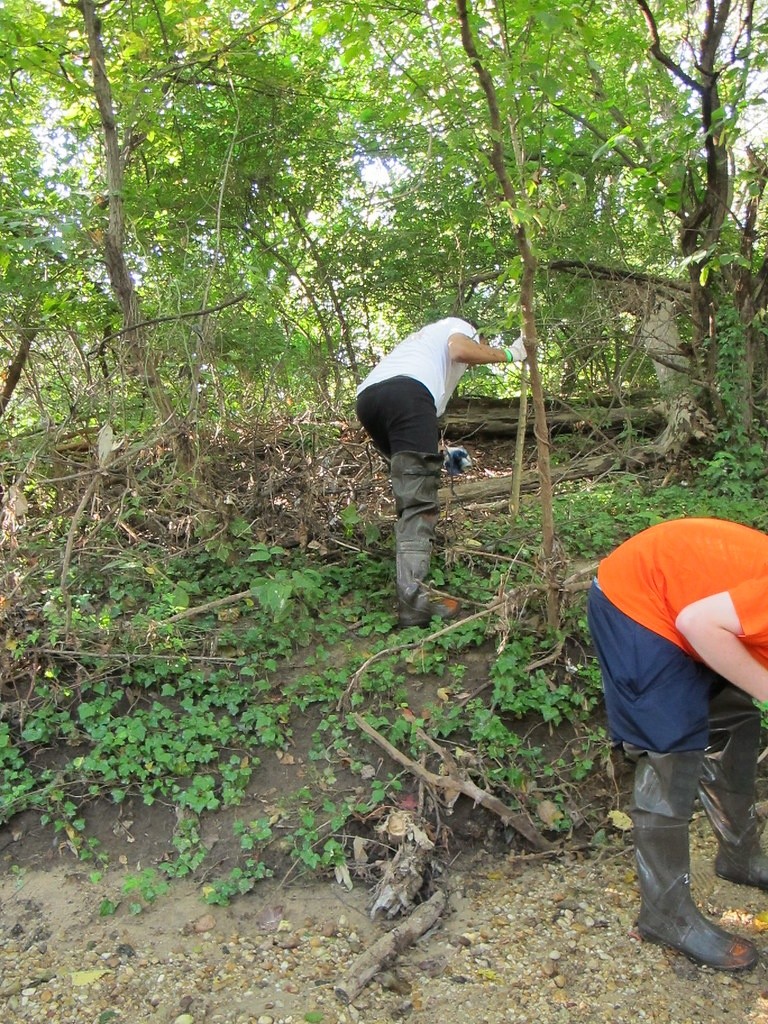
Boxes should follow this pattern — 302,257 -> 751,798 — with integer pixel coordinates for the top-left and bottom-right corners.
503,349 -> 513,363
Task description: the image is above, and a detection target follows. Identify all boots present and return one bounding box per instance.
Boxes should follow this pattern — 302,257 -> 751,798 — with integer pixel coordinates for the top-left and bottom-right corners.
391,449 -> 460,626
623,741 -> 757,971
700,689 -> 768,888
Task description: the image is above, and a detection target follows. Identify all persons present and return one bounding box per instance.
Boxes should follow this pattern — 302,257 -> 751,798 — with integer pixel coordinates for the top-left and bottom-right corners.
355,316 -> 528,629
586,518 -> 768,972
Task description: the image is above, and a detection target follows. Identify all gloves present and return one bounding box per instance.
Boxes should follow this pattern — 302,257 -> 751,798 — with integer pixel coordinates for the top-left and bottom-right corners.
505,335 -> 527,360
